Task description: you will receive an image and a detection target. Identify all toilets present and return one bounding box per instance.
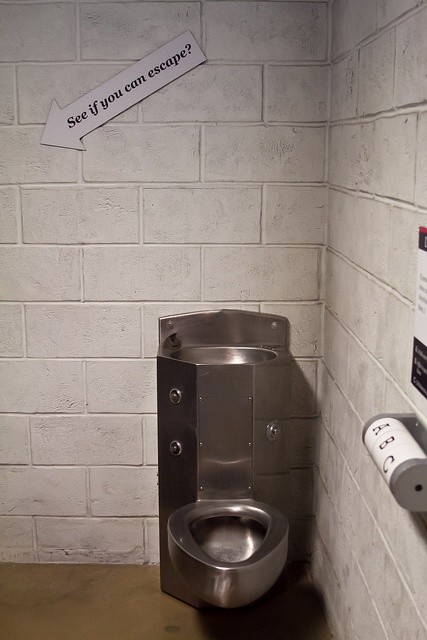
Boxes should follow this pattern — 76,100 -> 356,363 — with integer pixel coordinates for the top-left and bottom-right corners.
166,499 -> 291,611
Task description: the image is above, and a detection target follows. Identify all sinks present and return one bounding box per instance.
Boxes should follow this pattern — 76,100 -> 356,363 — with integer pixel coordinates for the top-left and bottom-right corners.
170,344 -> 280,365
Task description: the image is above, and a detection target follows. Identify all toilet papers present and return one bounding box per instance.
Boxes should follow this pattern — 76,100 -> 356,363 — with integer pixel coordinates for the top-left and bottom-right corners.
362,417 -> 427,486
